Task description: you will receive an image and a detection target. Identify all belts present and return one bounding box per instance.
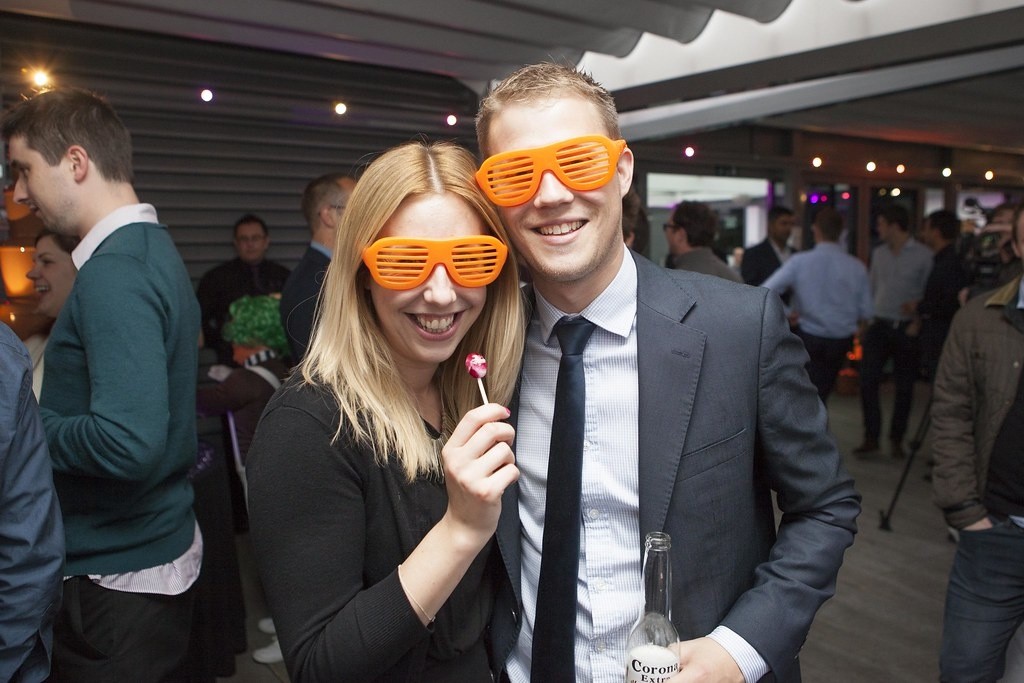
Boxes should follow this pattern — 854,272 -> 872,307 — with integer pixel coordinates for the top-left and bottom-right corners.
877,318 -> 907,332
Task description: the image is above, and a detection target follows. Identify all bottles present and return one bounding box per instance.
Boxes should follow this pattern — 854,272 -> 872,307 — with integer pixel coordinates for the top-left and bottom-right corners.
624,532 -> 682,683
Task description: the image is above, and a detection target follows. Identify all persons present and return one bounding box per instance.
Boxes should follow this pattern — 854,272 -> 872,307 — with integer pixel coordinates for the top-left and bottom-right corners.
931,203 -> 1024,683
251,616 -> 285,664
276,171 -> 359,363
620,186 -> 639,249
472,56 -> 858,683
661,197 -> 742,283
731,201 -> 1016,466
198,216 -> 288,369
0,86 -> 242,683
248,133 -> 530,683
0,319 -> 64,683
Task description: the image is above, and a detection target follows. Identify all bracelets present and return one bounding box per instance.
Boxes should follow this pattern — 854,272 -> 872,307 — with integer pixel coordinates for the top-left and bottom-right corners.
397,564 -> 436,622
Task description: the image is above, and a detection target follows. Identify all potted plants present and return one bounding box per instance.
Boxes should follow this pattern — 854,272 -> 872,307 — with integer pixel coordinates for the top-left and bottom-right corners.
220,294 -> 293,366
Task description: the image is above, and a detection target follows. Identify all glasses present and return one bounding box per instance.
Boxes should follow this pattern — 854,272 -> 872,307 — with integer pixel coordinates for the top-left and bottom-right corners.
662,221 -> 678,231
236,233 -> 268,245
357,234 -> 509,293
472,135 -> 628,210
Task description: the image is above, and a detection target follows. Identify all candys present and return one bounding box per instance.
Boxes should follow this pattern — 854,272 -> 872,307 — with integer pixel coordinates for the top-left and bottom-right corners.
465,352 -> 487,379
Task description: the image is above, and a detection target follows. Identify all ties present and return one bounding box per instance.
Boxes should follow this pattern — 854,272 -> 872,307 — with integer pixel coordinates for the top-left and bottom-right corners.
528,315 -> 596,683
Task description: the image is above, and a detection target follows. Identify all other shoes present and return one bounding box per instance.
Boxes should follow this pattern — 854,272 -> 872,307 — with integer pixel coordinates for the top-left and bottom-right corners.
852,439 -> 882,456
892,441 -> 906,458
